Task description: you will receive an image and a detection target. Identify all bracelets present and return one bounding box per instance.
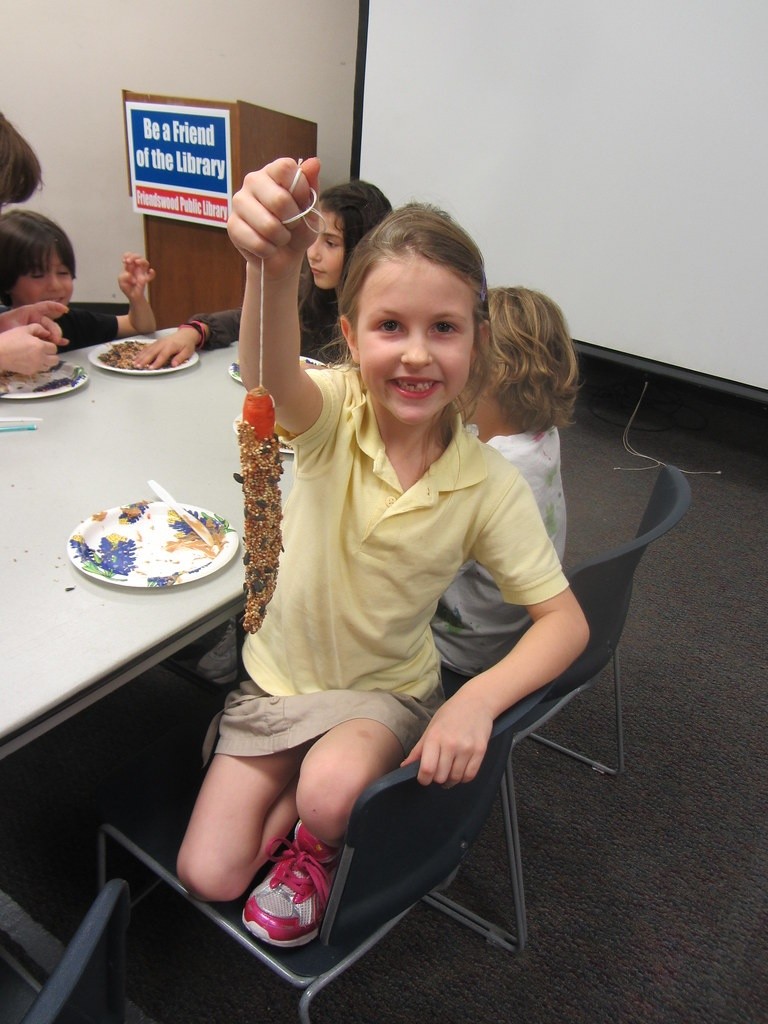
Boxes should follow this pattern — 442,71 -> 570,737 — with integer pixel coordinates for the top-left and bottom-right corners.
187,315 -> 205,348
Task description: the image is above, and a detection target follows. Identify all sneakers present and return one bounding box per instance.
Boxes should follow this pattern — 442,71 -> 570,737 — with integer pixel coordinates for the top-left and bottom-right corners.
241,818 -> 345,948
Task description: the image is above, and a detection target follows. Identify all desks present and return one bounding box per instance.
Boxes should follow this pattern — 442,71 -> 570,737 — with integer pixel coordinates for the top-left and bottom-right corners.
0,327 -> 337,763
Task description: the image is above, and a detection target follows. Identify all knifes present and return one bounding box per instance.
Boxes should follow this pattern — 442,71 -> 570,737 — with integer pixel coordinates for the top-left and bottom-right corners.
0,415 -> 43,422
148,479 -> 215,547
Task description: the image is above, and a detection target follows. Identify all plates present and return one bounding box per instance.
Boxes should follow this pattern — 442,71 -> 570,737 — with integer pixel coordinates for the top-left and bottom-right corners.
65,501 -> 239,588
232,411 -> 295,454
0,357 -> 89,400
88,339 -> 200,376
228,355 -> 326,383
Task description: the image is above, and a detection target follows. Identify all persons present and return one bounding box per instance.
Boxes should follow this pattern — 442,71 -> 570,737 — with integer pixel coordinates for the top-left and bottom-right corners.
0,112 -> 156,378
430,285 -> 579,702
128,180 -> 394,369
178,157 -> 591,947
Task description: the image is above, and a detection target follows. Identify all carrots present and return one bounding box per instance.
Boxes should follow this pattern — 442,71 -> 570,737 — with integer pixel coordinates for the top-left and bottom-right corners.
242,386 -> 275,441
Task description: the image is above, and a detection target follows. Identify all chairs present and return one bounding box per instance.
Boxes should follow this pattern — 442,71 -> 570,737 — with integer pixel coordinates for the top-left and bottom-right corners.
0,877 -> 155,1024
97,665 -> 556,1024
409,462 -> 690,953
